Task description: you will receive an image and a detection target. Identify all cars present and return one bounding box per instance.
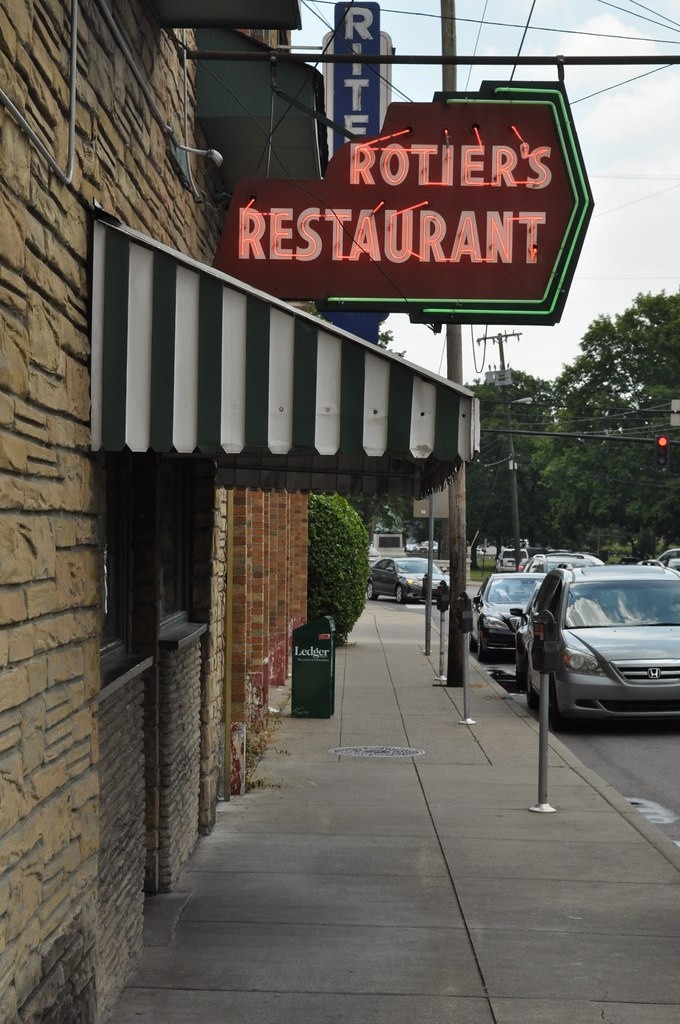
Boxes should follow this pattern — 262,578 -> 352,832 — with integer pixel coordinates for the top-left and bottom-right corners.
469,548 -> 680,692
368,540 -> 438,567
366,558 -> 450,604
526,559 -> 680,734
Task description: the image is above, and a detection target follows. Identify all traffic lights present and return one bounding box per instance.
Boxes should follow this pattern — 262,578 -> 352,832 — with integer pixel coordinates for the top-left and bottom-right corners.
657,437 -> 668,466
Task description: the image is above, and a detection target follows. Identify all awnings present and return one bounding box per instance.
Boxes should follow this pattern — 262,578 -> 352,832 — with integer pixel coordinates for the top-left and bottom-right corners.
88,202 -> 479,500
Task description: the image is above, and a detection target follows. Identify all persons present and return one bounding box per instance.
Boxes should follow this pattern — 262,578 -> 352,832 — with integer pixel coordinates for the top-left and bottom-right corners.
521,541 -> 526,548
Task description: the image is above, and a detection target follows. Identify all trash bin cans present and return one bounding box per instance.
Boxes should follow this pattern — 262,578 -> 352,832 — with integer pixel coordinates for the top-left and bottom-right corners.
292,615 -> 335,718
